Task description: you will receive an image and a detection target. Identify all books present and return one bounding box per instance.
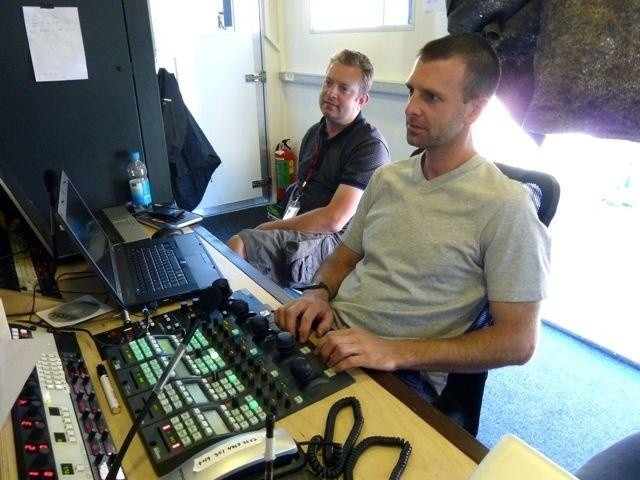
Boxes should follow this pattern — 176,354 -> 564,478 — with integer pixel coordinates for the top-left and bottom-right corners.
132,205 -> 203,235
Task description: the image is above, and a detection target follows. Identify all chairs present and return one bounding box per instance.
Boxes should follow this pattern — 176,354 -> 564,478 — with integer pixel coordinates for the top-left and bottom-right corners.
432,160 -> 561,440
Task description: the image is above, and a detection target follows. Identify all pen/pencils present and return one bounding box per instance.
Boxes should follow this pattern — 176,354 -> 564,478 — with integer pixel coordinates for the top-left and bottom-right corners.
96,364 -> 122,414
265,412 -> 275,480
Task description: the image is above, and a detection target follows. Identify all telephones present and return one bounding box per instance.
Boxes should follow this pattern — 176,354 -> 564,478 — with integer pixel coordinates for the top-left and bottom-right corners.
160,427 -> 298,480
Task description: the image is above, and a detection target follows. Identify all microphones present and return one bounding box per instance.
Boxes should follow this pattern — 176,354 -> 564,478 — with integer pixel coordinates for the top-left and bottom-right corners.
105,287 -> 221,480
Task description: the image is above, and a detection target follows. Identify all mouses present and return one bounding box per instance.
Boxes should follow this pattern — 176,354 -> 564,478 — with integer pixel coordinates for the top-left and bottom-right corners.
152,226 -> 184,239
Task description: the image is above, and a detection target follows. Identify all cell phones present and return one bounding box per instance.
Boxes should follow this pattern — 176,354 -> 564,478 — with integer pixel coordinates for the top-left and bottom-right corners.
147,206 -> 186,219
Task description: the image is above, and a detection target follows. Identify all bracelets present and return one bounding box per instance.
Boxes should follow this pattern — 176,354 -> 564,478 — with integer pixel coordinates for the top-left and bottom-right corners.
301,281 -> 333,301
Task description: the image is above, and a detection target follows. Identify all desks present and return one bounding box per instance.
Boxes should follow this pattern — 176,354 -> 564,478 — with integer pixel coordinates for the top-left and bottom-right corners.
0,215 -> 491,480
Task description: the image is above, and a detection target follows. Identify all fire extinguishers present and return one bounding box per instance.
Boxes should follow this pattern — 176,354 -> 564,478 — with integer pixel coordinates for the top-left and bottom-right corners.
273,139 -> 298,204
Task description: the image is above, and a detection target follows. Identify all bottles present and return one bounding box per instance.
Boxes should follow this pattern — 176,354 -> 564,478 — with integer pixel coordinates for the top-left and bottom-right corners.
127,152 -> 153,215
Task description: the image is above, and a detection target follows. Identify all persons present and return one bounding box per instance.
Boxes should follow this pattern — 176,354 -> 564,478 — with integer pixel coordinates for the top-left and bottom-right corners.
269,30 -> 555,405
224,48 -> 392,288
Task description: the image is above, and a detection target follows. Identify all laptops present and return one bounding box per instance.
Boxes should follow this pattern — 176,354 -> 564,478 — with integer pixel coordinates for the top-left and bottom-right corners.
53,168 -> 226,312
